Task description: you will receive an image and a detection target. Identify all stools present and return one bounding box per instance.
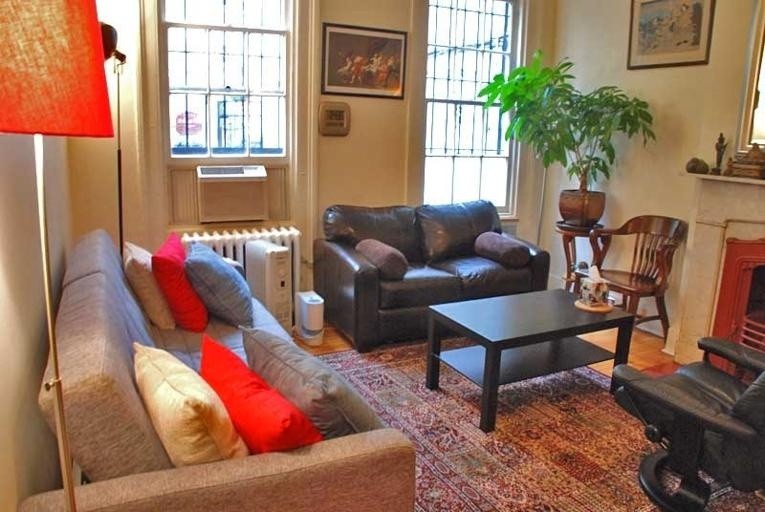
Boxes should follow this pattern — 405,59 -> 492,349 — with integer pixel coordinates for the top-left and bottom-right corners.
556,231 -> 589,293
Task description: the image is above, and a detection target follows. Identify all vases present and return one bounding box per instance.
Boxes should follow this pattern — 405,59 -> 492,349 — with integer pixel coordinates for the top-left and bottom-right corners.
322,24 -> 406,99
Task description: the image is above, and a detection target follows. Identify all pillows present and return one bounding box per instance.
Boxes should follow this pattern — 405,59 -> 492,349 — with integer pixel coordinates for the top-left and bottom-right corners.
126,243 -> 177,332
240,324 -> 387,438
475,231 -> 530,269
181,241 -> 255,332
355,238 -> 408,280
150,230 -> 210,334
197,333 -> 320,456
133,342 -> 247,468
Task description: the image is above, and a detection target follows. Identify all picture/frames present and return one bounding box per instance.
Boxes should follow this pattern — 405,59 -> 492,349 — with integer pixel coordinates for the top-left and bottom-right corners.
628,1 -> 714,71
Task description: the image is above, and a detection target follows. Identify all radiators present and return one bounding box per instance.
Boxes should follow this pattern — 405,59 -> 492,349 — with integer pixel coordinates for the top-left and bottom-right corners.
181,227 -> 301,331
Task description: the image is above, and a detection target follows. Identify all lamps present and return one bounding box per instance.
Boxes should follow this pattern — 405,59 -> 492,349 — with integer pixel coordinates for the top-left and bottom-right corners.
0,0 -> 115,512
97,22 -> 126,66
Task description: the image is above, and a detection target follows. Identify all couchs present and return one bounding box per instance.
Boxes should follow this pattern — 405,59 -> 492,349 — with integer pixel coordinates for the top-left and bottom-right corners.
23,231 -> 415,512
315,202 -> 552,353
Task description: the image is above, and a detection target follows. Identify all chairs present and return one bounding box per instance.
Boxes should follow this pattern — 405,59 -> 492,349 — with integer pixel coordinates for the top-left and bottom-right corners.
590,216 -> 687,344
610,335 -> 765,511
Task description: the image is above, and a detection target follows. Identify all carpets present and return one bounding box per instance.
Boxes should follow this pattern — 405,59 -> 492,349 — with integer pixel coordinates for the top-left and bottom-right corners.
317,338 -> 678,512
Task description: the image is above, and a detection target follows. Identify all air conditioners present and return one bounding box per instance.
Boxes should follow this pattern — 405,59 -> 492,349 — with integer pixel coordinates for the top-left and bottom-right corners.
197,164 -> 268,225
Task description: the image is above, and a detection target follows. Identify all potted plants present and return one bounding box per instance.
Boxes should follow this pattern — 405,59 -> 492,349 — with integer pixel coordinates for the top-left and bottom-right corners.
478,49 -> 655,227
